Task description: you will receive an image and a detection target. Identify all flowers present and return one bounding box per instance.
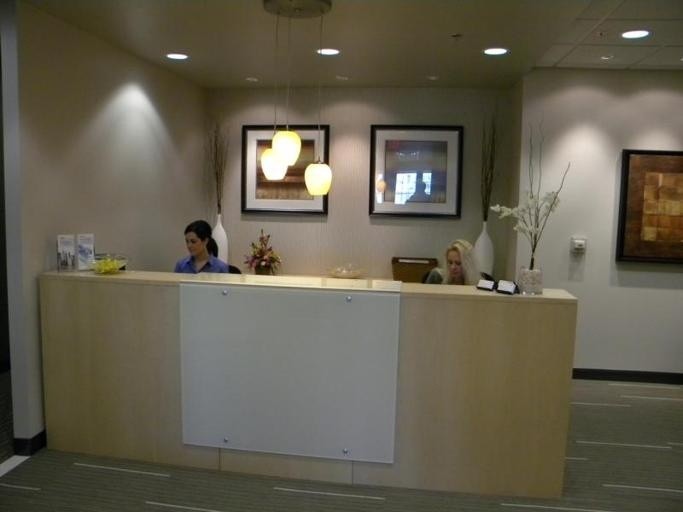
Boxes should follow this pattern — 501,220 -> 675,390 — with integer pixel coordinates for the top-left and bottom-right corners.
490,109 -> 573,269
242,228 -> 282,275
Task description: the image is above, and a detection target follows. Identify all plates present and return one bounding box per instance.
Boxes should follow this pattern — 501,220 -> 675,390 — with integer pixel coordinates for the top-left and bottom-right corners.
325,268 -> 366,278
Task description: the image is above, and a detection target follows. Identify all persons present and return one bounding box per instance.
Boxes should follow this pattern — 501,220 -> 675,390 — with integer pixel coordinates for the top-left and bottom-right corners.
426,240 -> 480,284
175,220 -> 230,272
407,180 -> 430,202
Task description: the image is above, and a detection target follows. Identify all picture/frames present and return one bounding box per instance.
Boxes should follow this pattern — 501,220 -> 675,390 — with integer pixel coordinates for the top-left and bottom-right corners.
240,123 -> 330,216
369,123 -> 463,219
615,149 -> 683,265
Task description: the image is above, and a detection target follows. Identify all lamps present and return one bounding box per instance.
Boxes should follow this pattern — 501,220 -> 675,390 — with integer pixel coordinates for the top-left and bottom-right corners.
260,2 -> 333,196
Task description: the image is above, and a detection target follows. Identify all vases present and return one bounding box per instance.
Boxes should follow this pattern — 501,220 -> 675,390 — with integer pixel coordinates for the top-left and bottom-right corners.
515,265 -> 543,294
472,219 -> 494,278
255,258 -> 269,275
211,214 -> 228,264
